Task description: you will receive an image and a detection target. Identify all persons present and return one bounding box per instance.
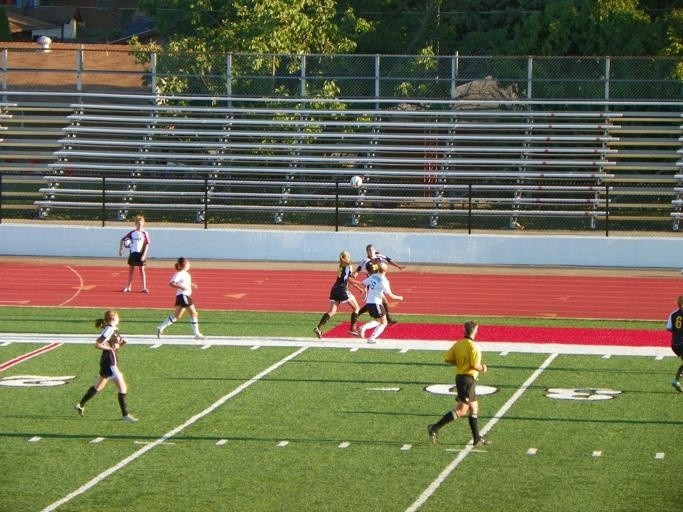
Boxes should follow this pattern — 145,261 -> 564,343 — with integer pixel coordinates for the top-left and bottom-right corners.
665,295 -> 683,391
426,320 -> 492,446
155,257 -> 203,339
352,244 -> 406,326
312,250 -> 361,339
72,310 -> 138,423
118,215 -> 149,292
346,263 -> 403,344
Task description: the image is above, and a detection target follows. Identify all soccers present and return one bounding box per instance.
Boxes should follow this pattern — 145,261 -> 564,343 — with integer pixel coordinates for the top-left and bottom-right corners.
351,174 -> 361,188
123,238 -> 131,248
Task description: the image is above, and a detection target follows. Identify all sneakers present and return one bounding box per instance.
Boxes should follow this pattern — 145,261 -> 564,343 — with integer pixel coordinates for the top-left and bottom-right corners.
428,424 -> 436,445
143,288 -> 149,292
195,334 -> 207,340
314,320 -> 397,345
157,327 -> 163,338
672,379 -> 683,392
122,415 -> 138,424
75,403 -> 85,418
122,287 -> 132,291
473,438 -> 491,448
